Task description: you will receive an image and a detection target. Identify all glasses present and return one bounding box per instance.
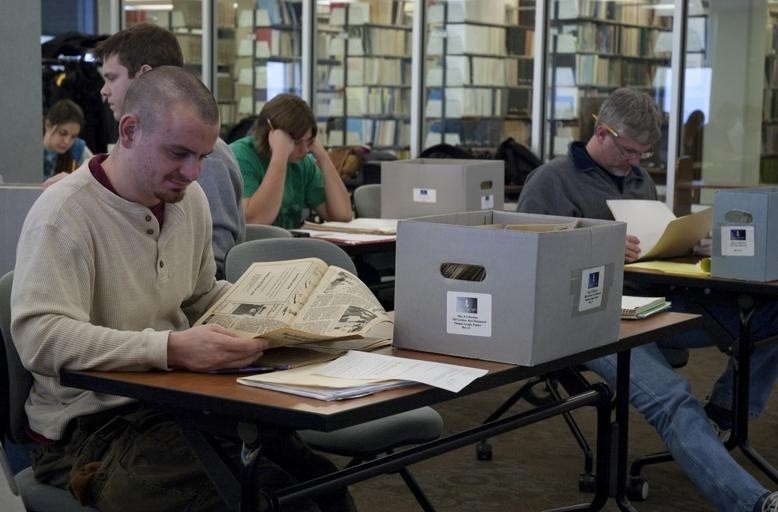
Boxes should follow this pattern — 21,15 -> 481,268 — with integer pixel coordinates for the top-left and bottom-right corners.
613,139 -> 654,159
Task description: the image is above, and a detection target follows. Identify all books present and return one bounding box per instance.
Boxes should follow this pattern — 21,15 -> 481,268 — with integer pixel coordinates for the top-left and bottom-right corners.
301,216 -> 403,237
232,353 -> 420,402
604,196 -> 714,263
119,2 -> 778,187
442,219 -> 597,286
622,292 -> 672,321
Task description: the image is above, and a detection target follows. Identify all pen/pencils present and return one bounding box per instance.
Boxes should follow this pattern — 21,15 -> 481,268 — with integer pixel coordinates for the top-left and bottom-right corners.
72,159 -> 76,172
207,364 -> 294,375
267,118 -> 274,130
591,112 -> 618,137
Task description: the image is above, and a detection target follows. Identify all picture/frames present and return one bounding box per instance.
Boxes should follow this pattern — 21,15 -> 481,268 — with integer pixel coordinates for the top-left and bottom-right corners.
686,15 -> 707,53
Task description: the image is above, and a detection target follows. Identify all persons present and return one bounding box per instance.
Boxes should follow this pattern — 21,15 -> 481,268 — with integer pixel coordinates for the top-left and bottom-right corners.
514,87 -> 777,509
7,63 -> 368,512
43,95 -> 97,184
225,90 -> 359,235
93,20 -> 244,283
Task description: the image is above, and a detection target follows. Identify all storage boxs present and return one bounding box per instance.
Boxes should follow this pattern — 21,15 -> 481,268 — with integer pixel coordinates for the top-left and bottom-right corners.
711,185 -> 778,282
379,157 -> 505,218
